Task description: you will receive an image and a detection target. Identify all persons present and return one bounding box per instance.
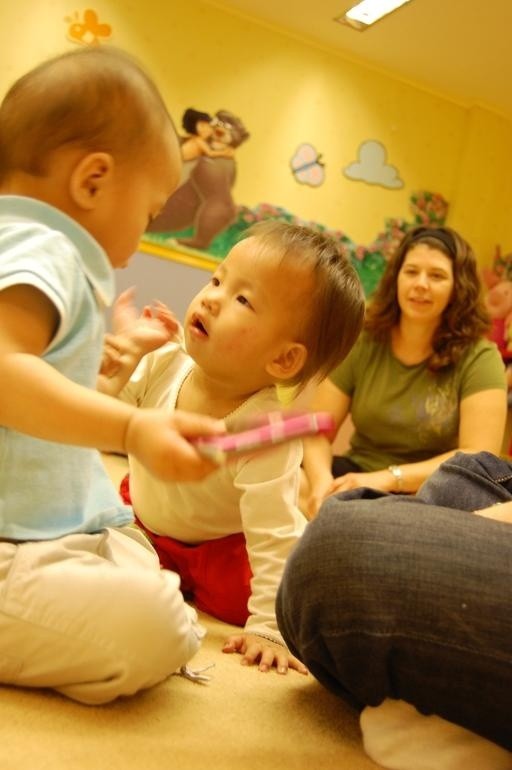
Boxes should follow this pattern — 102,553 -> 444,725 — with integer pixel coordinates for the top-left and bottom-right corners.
0,43 -> 226,707
268,449 -> 512,770
98,216 -> 361,674
298,226 -> 509,514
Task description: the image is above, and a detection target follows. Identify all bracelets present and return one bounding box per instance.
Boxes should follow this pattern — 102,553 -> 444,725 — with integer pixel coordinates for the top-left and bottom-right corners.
388,464 -> 403,494
